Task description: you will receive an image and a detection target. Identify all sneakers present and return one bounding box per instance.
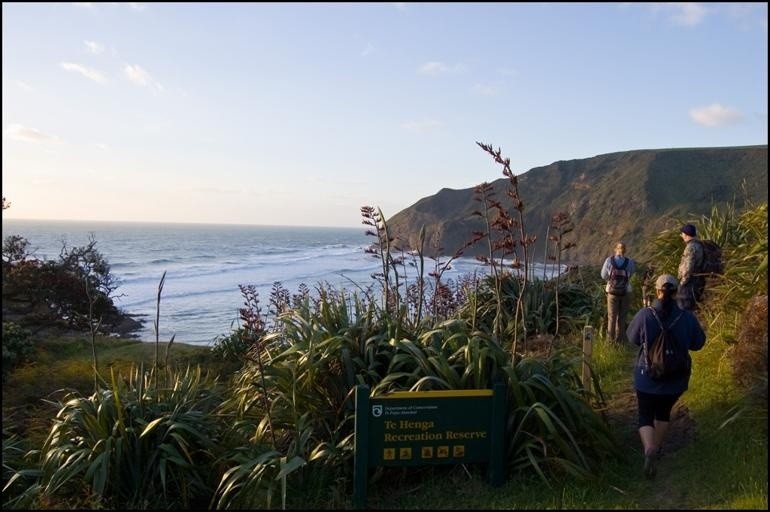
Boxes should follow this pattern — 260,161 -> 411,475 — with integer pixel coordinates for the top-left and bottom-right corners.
644,440 -> 665,480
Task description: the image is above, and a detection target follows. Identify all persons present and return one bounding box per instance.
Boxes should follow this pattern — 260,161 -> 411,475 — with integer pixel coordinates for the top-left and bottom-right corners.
598,241 -> 635,339
627,271 -> 706,477
676,225 -> 706,309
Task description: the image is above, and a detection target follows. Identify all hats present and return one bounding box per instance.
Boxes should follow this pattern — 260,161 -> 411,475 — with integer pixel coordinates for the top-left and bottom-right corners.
679,223 -> 696,237
654,273 -> 678,290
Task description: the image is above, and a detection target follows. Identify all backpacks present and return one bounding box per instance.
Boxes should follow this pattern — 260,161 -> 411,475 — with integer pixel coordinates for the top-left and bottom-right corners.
609,255 -> 629,296
643,306 -> 692,384
687,240 -> 722,279
